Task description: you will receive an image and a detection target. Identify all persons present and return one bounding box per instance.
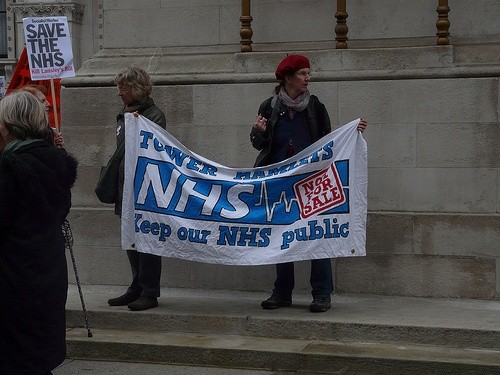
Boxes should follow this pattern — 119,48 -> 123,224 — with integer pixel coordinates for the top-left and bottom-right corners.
0,87 -> 79,375
249,55 -> 368,313
106,66 -> 166,310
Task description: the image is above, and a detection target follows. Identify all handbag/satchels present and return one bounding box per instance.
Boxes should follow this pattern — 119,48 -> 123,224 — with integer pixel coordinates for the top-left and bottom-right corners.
93,163 -> 120,204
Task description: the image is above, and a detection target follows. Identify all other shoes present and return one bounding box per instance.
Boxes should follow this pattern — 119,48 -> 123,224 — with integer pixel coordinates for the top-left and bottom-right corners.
127,297 -> 159,311
107,292 -> 138,306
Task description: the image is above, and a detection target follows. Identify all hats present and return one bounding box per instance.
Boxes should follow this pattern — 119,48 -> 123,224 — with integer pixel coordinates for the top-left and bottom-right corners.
274,52 -> 311,80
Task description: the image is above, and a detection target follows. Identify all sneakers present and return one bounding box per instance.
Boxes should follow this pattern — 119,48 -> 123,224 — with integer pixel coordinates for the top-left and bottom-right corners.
261,293 -> 292,309
308,294 -> 331,313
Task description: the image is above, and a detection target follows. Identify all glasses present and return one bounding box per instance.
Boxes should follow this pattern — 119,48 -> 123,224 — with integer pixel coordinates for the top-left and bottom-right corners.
117,87 -> 132,93
293,71 -> 312,78
